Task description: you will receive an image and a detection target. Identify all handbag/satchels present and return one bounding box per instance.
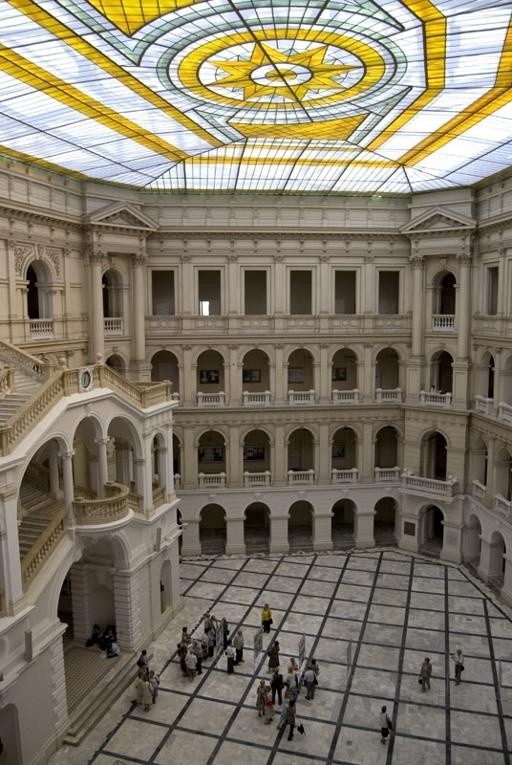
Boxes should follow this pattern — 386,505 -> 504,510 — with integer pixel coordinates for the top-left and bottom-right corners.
314,675 -> 318,685
279,712 -> 288,720
269,618 -> 273,624
418,679 -> 422,684
460,665 -> 464,671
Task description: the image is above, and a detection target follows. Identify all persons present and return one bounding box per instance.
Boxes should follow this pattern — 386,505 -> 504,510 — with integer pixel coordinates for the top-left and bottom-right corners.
261,603 -> 273,634
267,640 -> 280,674
380,705 -> 392,744
253,658 -> 320,740
91,624 -> 120,658
420,658 -> 432,692
451,649 -> 464,685
130,650 -> 159,712
176,613 -> 245,681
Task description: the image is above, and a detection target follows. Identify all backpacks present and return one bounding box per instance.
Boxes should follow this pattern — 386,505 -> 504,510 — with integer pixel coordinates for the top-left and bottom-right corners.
386,713 -> 393,729
